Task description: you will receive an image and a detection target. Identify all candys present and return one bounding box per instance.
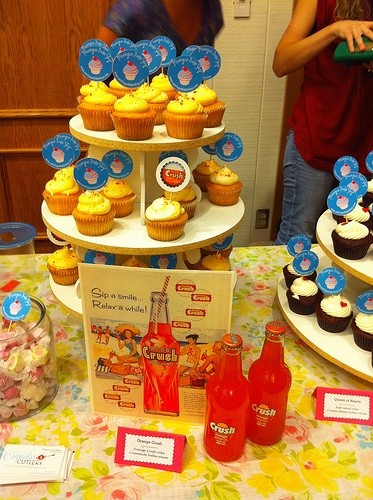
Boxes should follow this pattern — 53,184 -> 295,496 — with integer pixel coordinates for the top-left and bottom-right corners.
0,308 -> 57,420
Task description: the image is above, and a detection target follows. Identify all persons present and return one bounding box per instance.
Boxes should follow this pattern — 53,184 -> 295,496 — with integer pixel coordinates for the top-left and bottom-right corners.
272,0 -> 373,245
97,0 -> 225,87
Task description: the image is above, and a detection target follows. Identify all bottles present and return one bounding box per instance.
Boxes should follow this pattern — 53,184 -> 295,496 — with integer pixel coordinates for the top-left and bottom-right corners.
203,333 -> 248,462
141,290 -> 179,417
246,320 -> 292,446
0,294 -> 61,423
0,222 -> 37,296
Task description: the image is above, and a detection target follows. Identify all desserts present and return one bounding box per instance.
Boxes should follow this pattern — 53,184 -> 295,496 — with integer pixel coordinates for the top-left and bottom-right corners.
41,159 -> 243,242
330,177 -> 373,260
76,73 -> 227,141
47,245 -> 234,286
283,260 -> 373,354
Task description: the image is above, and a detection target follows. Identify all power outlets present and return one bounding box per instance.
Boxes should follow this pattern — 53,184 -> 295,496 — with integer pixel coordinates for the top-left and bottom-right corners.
255,208 -> 270,230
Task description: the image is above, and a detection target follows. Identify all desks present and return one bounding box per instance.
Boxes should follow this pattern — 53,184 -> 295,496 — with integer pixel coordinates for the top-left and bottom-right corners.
0,243 -> 373,500
